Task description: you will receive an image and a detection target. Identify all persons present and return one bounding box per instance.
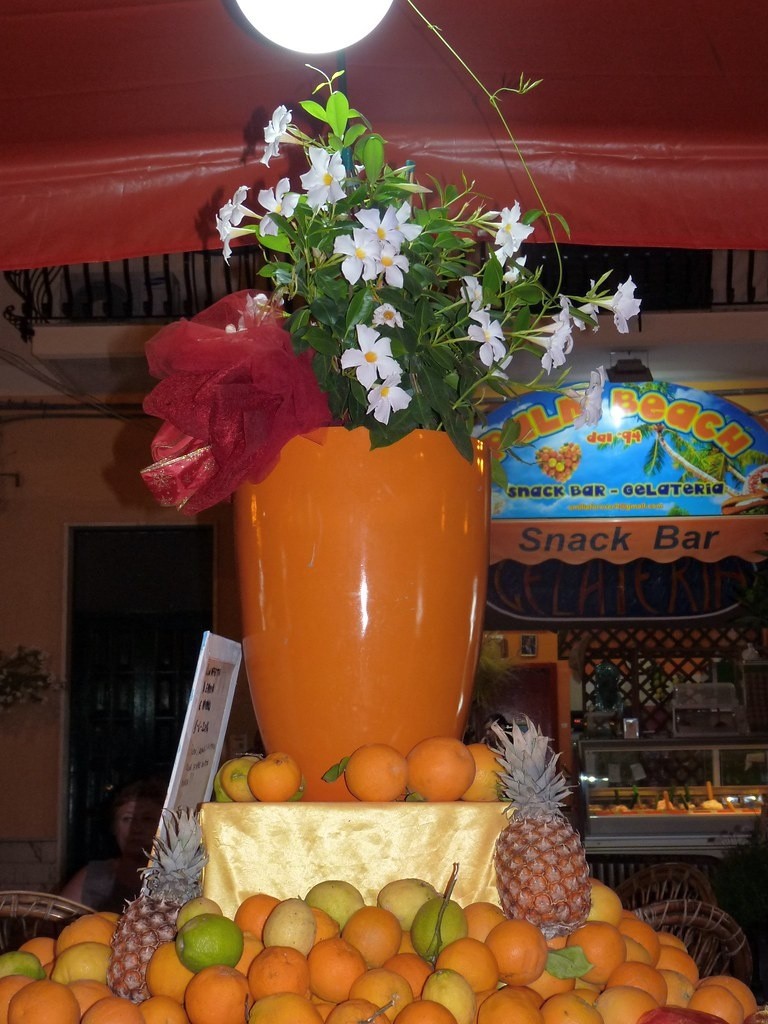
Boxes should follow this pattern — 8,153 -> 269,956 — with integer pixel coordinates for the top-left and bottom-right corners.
483,712 -> 529,753
55,775 -> 170,915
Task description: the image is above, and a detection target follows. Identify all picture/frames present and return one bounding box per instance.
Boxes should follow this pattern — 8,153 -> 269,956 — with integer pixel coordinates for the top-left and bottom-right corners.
520,634 -> 538,657
623,718 -> 639,738
488,634 -> 505,657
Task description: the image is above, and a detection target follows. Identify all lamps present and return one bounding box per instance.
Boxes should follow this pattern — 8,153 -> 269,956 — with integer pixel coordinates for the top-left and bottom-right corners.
606,345 -> 654,382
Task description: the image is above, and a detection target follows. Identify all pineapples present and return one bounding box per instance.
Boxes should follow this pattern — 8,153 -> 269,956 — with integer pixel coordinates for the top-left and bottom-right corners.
484,712 -> 591,936
106,809 -> 211,1005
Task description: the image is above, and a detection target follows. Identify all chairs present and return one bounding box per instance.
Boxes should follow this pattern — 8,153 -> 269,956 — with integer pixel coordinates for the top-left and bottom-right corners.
0,890 -> 94,957
616,863 -> 754,988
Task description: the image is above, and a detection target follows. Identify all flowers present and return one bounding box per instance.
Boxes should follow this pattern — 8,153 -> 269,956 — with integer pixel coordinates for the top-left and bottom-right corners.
216,1 -> 643,461
0,643 -> 65,713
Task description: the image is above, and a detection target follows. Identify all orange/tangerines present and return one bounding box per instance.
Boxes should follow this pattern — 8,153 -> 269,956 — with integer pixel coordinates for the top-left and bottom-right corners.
211,734 -> 507,802
0,879 -> 768,1024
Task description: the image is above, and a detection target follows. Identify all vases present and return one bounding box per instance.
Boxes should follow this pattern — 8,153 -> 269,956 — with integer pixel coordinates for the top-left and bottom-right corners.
233,428 -> 491,801
